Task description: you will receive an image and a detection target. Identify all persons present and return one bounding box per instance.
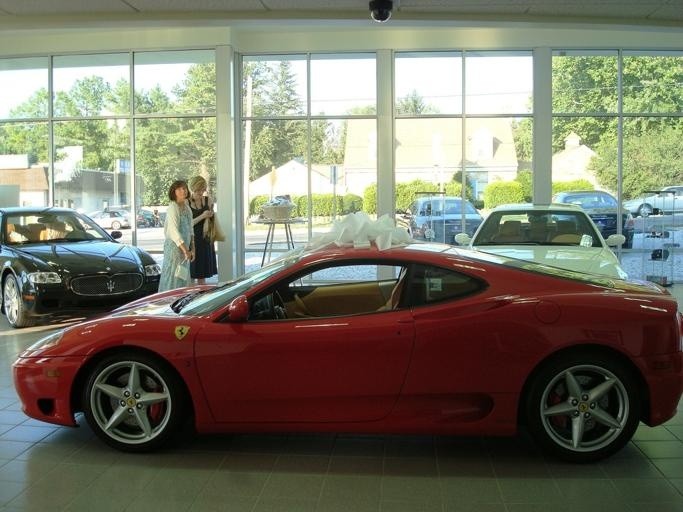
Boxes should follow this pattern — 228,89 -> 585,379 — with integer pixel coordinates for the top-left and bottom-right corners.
187,175 -> 218,285
158,181 -> 196,292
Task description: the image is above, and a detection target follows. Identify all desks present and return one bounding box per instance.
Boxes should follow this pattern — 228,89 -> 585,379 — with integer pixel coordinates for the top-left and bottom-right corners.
251,219 -> 305,288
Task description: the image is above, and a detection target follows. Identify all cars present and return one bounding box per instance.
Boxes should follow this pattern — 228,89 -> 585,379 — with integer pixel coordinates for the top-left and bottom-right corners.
453,202 -> 626,279
552,190 -> 635,248
80,210 -> 129,230
404,196 -> 484,244
623,185 -> 683,217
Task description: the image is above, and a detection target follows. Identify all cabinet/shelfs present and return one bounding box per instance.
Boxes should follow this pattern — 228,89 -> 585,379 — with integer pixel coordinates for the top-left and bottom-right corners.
414,192 -> 445,244
644,191 -> 676,287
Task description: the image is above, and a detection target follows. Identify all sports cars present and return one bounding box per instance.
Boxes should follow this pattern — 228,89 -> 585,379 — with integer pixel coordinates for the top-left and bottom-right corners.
11,241 -> 683,464
0,206 -> 160,327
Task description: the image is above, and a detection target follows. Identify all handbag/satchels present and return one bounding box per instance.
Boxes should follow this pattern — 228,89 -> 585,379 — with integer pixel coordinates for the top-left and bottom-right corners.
173,258 -> 190,281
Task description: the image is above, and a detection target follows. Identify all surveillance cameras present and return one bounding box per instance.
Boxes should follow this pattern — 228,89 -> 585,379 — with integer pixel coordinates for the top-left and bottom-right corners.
368,0 -> 394,23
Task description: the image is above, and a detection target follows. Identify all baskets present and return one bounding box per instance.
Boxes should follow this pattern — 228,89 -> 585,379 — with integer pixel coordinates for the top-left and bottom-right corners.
260,205 -> 292,220
